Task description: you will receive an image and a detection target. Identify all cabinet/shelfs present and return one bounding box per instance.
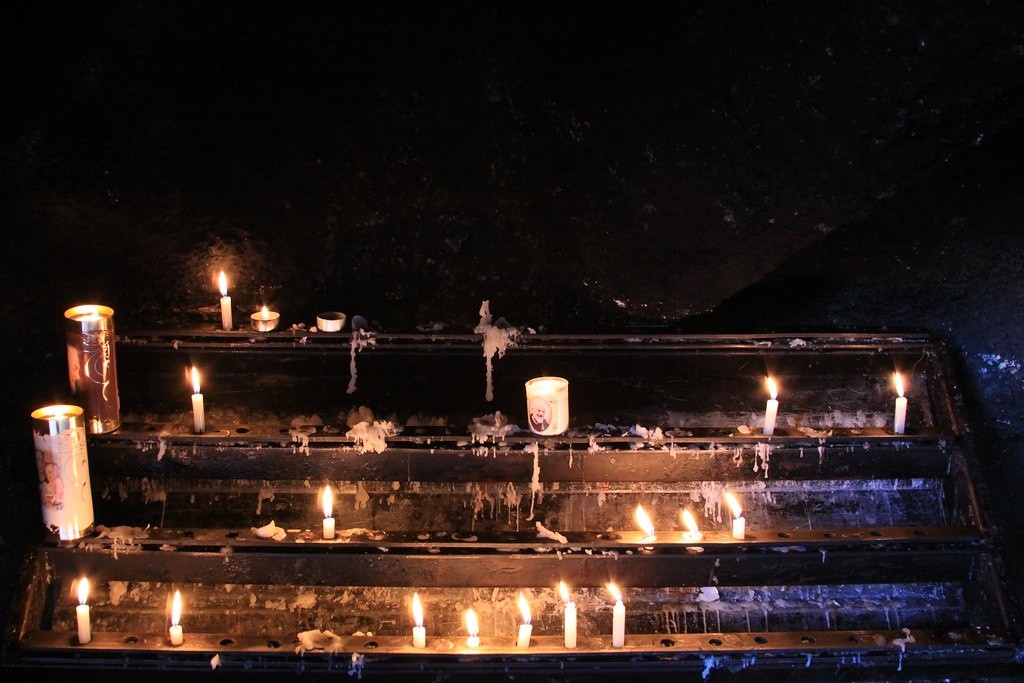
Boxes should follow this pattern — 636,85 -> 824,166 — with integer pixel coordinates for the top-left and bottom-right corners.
0,320 -> 1024,683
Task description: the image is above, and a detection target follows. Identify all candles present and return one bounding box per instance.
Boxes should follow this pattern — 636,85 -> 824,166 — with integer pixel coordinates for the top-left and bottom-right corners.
724,489 -> 745,540
525,374 -> 569,437
28,404 -> 94,539
557,580 -> 579,648
75,574 -> 93,645
315,311 -> 346,331
682,509 -> 702,541
169,589 -> 184,647
463,607 -> 481,647
251,304 -> 280,332
187,363 -> 206,431
761,371 -> 905,435
517,590 -> 533,646
411,591 -> 426,650
321,485 -> 335,538
606,583 -> 626,646
635,502 -> 657,541
218,271 -> 233,329
65,303 -> 119,435
